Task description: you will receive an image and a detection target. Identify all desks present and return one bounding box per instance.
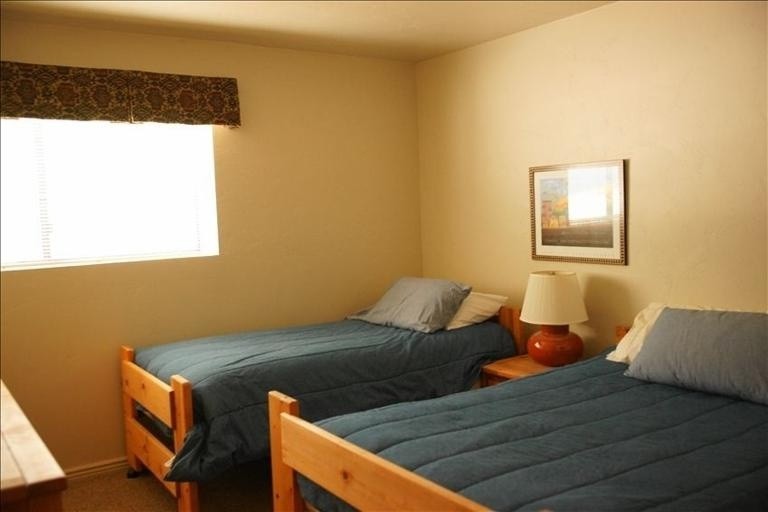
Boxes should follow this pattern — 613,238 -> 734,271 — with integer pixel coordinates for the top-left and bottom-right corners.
0,379 -> 68,512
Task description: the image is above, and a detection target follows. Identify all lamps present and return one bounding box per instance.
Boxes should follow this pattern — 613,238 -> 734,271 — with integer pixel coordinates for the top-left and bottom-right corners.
519,271 -> 589,367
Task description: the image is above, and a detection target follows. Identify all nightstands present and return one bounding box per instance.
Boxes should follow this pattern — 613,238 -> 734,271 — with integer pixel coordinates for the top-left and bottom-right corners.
480,354 -> 550,387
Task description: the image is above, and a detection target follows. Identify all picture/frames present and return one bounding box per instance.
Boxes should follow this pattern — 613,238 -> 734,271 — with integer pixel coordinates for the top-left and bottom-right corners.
529,159 -> 626,265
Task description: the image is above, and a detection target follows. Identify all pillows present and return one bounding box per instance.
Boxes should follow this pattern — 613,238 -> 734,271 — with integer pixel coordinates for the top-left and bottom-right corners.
605,303 -> 768,365
444,292 -> 509,331
623,307 -> 768,405
344,277 -> 472,333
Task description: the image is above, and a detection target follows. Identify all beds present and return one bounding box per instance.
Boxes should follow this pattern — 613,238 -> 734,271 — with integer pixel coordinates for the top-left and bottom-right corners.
120,306 -> 527,512
268,325 -> 768,512
